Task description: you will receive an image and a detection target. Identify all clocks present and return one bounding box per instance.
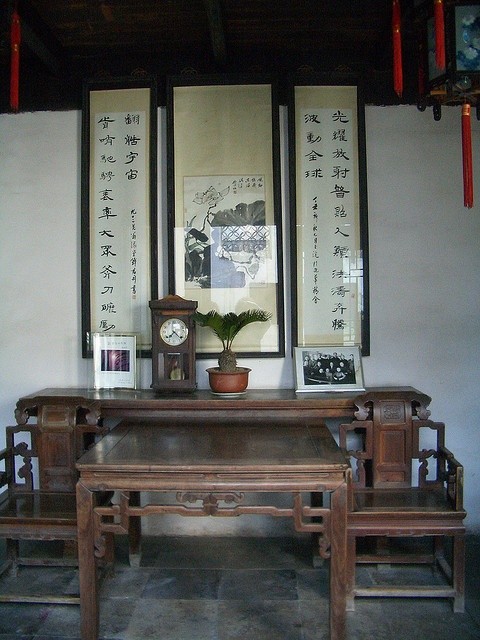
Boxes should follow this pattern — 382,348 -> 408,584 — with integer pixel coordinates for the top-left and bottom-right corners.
149,297 -> 199,393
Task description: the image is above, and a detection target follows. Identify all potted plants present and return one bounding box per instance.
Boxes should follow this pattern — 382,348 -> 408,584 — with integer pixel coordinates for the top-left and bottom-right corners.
193,310 -> 272,398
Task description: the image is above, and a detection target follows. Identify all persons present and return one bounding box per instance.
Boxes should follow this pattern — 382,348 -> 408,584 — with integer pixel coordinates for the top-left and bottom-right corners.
324,368 -> 332,380
348,354 -> 354,374
317,368 -> 325,380
332,367 -> 346,381
339,353 -> 349,367
303,353 -> 329,368
339,362 -> 348,375
330,362 -> 336,372
332,352 -> 340,366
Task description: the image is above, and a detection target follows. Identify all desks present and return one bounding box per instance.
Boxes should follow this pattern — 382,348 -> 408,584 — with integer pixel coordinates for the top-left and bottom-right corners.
13,388 -> 432,562
75,423 -> 352,639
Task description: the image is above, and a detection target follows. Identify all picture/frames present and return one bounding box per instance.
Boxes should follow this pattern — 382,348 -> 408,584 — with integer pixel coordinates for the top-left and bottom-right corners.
165,74 -> 286,357
294,344 -> 366,394
79,73 -> 156,360
285,77 -> 371,358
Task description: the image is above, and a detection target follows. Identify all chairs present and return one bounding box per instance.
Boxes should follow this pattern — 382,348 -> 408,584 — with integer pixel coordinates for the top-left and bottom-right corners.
339,390 -> 467,614
1,395 -> 115,607
